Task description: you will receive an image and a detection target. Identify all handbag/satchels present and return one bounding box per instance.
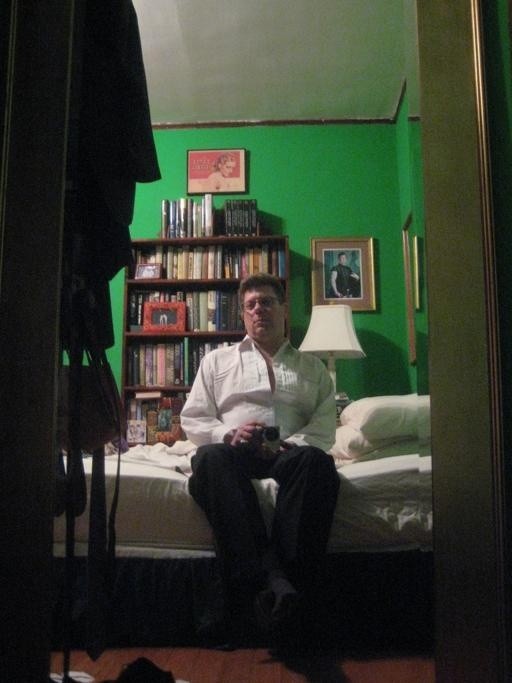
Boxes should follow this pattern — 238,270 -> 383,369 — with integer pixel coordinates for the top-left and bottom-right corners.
75,358 -> 128,456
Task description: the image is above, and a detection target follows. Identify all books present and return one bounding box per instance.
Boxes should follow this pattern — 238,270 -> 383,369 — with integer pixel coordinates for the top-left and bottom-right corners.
124,192 -> 285,431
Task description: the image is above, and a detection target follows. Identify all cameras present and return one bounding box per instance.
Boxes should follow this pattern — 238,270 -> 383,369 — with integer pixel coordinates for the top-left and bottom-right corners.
262,426 -> 279,453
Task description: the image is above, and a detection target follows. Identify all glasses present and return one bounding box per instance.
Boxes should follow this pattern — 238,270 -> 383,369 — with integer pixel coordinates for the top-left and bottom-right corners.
240,296 -> 280,310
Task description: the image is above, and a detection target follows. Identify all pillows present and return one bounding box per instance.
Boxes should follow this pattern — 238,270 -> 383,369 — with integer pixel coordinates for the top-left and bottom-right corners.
334,393 -> 431,460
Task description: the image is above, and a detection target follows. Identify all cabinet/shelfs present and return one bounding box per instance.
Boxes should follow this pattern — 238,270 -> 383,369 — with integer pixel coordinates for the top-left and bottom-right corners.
121,235 -> 289,445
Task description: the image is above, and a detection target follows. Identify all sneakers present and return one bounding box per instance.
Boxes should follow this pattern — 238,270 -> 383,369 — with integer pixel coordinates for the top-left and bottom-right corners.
104,656 -> 175,683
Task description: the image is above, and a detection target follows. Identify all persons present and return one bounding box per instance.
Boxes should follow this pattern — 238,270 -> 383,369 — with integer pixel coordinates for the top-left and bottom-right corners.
330,252 -> 360,298
202,153 -> 236,191
180,272 -> 341,652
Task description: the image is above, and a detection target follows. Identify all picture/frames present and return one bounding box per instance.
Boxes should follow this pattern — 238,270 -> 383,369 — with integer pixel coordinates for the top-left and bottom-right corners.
311,237 -> 377,313
134,263 -> 161,279
187,150 -> 247,195
142,302 -> 186,334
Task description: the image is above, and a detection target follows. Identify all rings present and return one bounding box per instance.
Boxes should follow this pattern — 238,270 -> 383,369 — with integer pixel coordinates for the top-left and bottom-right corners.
240,439 -> 245,445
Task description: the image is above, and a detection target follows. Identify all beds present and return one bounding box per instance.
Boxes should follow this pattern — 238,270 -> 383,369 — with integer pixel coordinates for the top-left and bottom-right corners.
54,452 -> 432,650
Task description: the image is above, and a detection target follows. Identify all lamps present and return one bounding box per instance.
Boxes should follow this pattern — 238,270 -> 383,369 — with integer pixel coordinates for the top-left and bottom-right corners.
297,305 -> 366,395
